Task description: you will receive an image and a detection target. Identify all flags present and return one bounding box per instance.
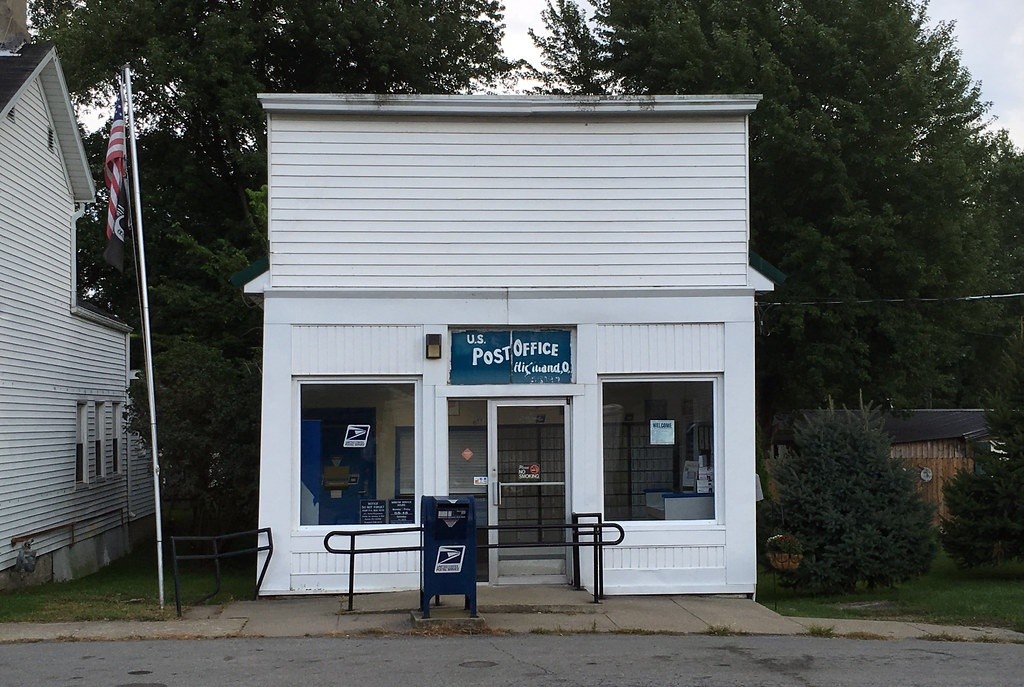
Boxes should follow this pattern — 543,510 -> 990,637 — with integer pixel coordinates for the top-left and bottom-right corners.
102,92 -> 128,272
